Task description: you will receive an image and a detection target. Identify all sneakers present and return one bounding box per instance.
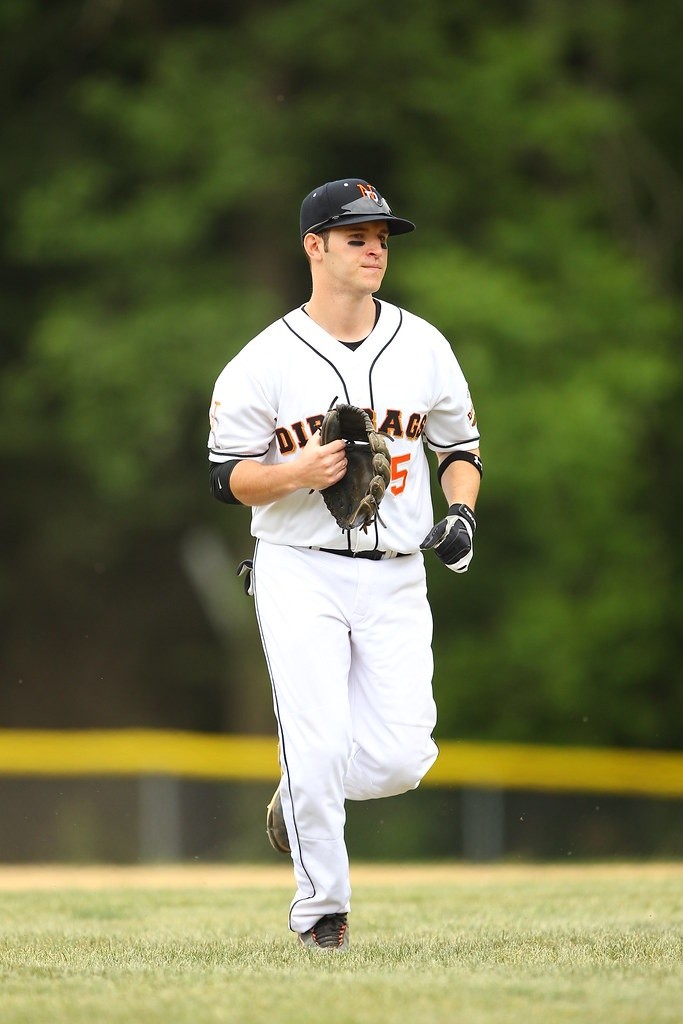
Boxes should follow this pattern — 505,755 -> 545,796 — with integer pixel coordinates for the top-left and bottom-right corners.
297,913 -> 350,957
266,780 -> 291,852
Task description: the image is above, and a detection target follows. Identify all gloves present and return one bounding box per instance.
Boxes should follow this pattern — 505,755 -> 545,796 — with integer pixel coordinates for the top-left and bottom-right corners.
420,502 -> 477,574
236,560 -> 255,597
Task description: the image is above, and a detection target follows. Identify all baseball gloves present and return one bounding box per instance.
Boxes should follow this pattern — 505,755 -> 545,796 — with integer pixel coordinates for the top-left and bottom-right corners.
320,403 -> 390,528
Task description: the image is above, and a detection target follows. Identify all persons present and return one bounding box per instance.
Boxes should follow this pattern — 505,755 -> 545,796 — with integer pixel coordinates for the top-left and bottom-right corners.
208,177 -> 483,943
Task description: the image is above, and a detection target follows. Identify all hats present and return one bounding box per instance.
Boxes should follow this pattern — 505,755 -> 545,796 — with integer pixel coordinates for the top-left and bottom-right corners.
300,178 -> 417,247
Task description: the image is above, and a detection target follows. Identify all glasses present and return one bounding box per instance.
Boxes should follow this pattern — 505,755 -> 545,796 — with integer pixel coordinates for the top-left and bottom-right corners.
303,196 -> 393,239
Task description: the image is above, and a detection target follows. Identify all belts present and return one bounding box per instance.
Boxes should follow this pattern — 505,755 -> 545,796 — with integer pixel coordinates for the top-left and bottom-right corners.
320,548 -> 407,562
